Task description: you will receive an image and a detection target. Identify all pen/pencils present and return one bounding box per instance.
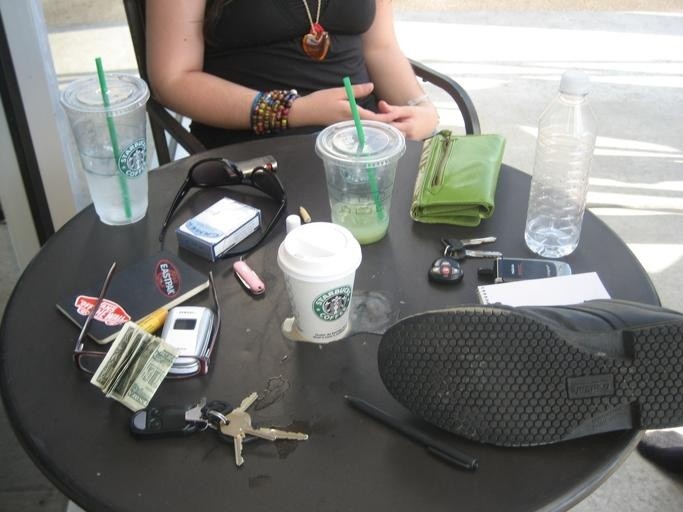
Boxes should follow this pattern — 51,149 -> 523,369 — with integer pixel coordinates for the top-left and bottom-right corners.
343,393 -> 479,470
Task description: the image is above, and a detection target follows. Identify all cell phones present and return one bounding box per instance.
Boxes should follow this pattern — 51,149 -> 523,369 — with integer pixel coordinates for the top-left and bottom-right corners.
161,305 -> 213,374
477,258 -> 571,284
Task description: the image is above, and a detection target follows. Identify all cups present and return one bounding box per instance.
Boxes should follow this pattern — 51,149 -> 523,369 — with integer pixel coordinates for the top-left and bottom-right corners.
314,119 -> 405,247
60,70 -> 151,228
275,223 -> 362,345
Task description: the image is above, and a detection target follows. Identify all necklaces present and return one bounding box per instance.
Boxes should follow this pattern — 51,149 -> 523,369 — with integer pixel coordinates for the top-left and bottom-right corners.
301,0 -> 331,63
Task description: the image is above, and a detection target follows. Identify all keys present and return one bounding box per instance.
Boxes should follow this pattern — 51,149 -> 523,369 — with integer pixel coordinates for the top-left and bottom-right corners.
130,390 -> 309,467
424,234 -> 502,283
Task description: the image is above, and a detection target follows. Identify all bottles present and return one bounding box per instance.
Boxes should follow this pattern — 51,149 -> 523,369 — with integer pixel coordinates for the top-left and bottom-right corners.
524,70 -> 596,258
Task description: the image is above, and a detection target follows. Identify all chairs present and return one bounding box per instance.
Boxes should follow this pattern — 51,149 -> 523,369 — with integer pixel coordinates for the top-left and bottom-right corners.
124,1 -> 481,167
0,135 -> 662,511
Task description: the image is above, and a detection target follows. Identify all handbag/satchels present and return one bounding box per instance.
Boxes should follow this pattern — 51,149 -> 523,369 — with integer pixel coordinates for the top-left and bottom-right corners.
410,130 -> 506,227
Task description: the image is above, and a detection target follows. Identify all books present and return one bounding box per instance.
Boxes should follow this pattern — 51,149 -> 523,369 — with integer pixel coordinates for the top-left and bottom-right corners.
474,272 -> 612,309
56,249 -> 211,345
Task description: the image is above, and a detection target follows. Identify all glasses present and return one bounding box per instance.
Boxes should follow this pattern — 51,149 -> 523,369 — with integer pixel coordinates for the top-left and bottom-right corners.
71,261 -> 221,380
159,157 -> 287,260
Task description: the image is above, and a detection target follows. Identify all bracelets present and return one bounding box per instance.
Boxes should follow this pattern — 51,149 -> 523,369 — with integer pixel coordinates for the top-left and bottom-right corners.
249,87 -> 300,135
405,93 -> 428,107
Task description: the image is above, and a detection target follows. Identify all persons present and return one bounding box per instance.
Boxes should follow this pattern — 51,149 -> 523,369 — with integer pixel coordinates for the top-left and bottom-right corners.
145,0 -> 443,143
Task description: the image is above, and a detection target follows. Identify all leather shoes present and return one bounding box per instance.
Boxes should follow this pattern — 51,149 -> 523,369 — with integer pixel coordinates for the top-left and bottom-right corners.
377,299 -> 682,447
